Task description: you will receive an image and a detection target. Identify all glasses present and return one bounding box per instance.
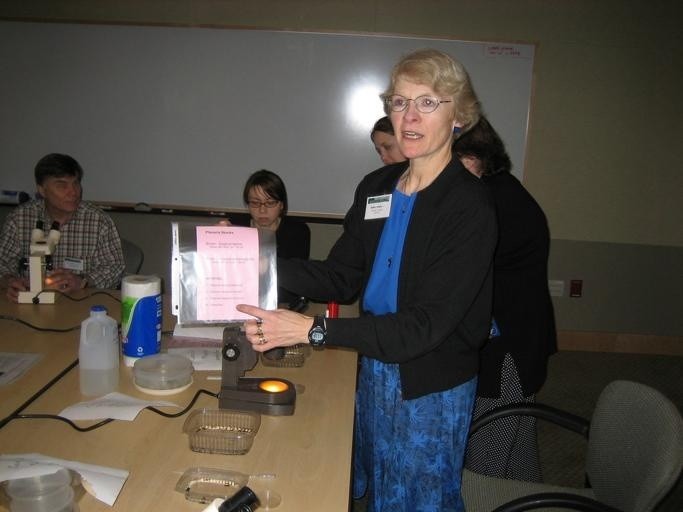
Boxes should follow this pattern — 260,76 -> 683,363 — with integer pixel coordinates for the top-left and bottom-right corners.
386,94 -> 451,113
248,201 -> 277,207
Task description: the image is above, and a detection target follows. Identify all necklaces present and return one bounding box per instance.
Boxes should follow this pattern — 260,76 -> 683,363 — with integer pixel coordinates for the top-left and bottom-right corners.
402,170 -> 422,213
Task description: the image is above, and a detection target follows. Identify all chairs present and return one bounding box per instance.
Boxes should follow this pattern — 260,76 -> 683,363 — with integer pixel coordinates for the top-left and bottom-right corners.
462,379 -> 683,511
120,237 -> 144,276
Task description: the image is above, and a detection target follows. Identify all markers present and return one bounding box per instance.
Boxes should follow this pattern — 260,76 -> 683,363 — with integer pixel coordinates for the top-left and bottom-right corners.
161,208 -> 175,216
97,204 -> 114,210
209,211 -> 225,216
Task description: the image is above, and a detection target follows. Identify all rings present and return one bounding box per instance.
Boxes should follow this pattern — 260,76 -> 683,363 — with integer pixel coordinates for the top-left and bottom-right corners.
257,327 -> 263,335
62,284 -> 67,288
259,335 -> 265,344
257,319 -> 263,327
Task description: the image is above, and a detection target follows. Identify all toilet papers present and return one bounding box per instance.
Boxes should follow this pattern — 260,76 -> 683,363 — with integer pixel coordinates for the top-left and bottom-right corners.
121,275 -> 162,367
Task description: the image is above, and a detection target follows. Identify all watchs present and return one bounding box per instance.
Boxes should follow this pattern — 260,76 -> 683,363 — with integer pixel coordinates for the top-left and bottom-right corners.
308,316 -> 326,347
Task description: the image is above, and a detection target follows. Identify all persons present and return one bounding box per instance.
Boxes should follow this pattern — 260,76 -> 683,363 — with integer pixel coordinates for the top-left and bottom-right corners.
228,172 -> 311,302
452,115 -> 556,484
219,48 -> 498,512
0,153 -> 126,303
370,116 -> 409,166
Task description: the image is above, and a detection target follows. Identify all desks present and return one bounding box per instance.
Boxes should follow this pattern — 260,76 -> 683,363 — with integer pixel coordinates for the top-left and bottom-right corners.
0,273 -> 361,512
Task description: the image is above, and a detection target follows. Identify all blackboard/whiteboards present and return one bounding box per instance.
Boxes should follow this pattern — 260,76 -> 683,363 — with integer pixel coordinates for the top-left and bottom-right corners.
0,16 -> 538,219
